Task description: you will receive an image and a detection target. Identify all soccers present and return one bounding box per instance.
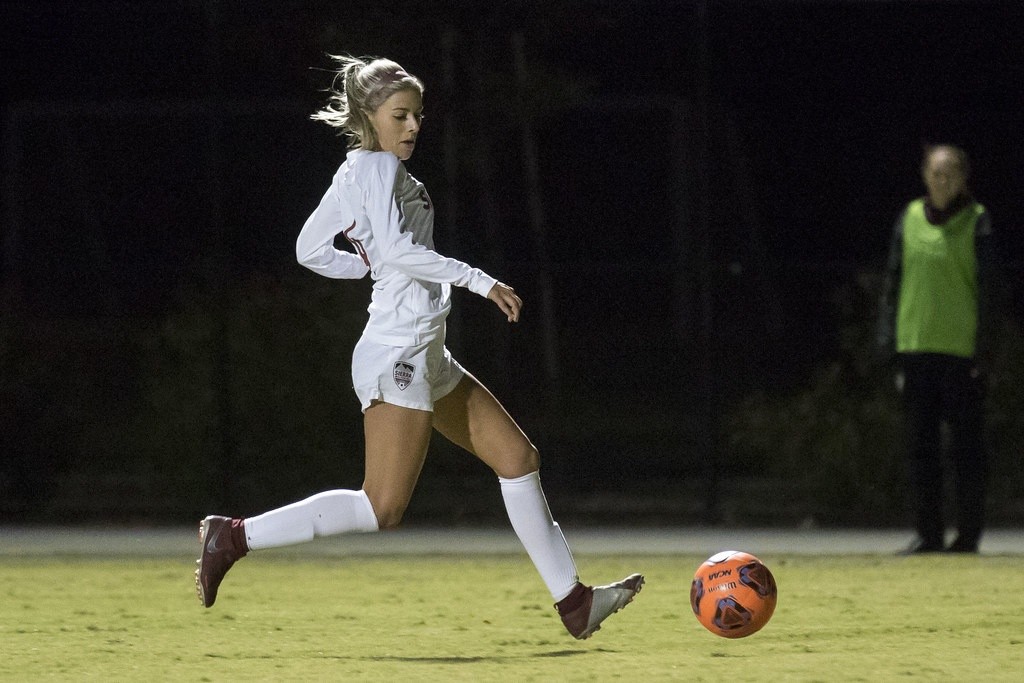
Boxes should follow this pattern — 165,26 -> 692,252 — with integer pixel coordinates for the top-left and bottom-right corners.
689,550 -> 779,640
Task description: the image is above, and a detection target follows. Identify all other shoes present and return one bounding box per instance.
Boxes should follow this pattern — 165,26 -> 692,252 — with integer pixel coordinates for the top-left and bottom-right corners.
897,536 -> 941,555
950,538 -> 976,551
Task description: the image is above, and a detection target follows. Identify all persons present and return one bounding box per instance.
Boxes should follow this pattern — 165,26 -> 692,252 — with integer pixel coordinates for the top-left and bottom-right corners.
877,147 -> 1002,553
194,58 -> 645,639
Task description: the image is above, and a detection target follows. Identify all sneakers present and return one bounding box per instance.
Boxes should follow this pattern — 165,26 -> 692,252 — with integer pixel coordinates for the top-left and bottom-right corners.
561,573 -> 645,641
194,515 -> 242,608
